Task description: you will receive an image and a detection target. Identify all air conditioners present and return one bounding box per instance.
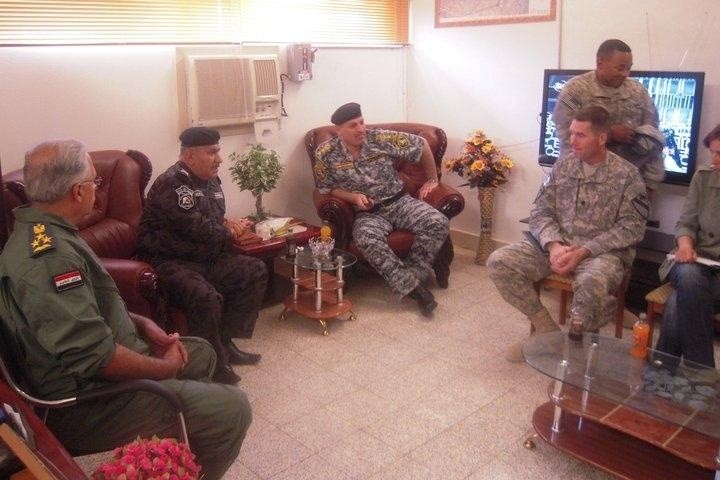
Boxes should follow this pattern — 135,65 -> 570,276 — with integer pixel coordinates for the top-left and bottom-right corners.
184,53 -> 282,143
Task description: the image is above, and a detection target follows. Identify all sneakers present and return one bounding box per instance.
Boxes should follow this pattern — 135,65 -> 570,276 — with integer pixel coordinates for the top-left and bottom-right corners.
413,286 -> 437,314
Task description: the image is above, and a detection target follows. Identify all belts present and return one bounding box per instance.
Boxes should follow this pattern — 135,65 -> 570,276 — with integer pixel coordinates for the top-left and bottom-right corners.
368,186 -> 406,213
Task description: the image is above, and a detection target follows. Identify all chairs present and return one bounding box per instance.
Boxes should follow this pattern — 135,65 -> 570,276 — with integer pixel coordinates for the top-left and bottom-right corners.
645,282 -> 720,362
305,122 -> 465,294
530,267 -> 629,339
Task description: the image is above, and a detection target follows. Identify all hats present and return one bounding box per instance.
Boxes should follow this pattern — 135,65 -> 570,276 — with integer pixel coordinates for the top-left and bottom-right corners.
331,102 -> 361,125
179,126 -> 220,146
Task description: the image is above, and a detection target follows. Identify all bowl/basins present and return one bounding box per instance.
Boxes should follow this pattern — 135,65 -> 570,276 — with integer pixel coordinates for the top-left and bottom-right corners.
308,236 -> 337,256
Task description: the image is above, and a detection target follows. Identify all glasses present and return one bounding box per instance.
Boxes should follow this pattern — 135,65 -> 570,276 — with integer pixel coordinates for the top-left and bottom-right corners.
80,176 -> 103,187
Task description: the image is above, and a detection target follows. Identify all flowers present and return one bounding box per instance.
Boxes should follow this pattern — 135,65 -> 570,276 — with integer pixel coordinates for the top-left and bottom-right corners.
444,128 -> 515,193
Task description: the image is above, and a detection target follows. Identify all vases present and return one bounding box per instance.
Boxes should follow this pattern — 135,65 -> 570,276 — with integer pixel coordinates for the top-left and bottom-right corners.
474,187 -> 498,267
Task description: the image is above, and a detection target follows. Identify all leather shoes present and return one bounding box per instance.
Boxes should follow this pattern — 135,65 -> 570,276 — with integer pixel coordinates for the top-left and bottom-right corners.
211,339 -> 262,386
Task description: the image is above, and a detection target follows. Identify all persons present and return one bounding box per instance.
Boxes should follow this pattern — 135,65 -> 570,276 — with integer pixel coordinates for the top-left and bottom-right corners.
485,107 -> 650,377
313,102 -> 449,315
133,126 -> 269,383
552,39 -> 659,330
0,139 -> 253,480
641,124 -> 720,393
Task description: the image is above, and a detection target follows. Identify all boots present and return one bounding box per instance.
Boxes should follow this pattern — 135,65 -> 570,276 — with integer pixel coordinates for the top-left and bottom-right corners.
505,307 -> 566,363
568,338 -> 609,376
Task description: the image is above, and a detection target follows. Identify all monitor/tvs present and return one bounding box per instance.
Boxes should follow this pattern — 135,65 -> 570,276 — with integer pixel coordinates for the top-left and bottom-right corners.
539,70 -> 704,187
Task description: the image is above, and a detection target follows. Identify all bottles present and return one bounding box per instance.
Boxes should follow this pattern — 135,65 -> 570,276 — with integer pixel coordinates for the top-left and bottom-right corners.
567,297 -> 585,342
629,312 -> 651,359
284,226 -> 297,258
320,219 -> 331,239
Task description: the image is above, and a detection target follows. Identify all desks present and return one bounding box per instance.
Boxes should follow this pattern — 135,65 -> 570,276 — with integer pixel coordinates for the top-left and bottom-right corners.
519,217 -> 676,291
277,246 -> 358,336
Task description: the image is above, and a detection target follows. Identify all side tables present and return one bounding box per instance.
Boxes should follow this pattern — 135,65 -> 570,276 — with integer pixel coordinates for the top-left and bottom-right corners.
231,215 -> 322,309
